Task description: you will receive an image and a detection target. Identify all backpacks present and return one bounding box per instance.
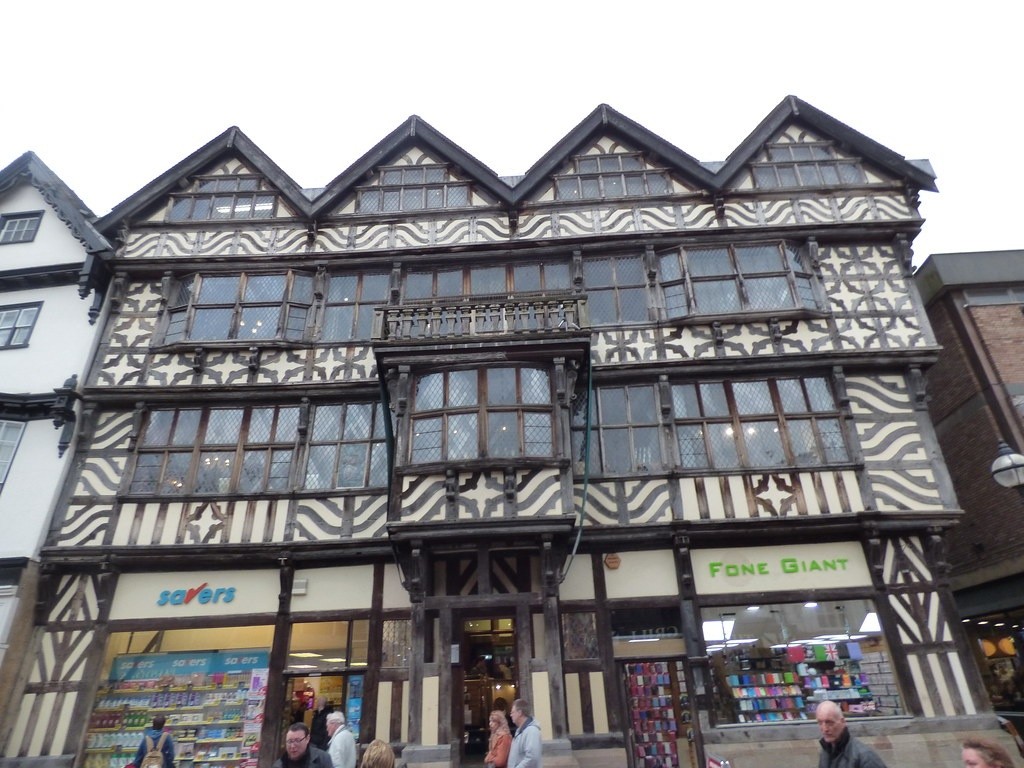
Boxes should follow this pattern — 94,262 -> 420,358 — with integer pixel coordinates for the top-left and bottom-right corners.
141,732 -> 168,768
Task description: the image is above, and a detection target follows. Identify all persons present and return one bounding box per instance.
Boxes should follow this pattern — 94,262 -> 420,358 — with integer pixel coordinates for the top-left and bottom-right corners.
309,694 -> 334,751
294,701 -> 309,722
506,699 -> 543,768
133,715 -> 174,768
326,711 -> 356,768
492,697 -> 514,736
960,735 -> 1014,768
272,722 -> 334,768
814,701 -> 888,768
360,739 -> 395,768
484,710 -> 513,768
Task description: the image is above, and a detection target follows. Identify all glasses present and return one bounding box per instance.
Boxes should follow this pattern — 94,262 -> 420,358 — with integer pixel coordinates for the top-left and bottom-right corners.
287,735 -> 307,744
